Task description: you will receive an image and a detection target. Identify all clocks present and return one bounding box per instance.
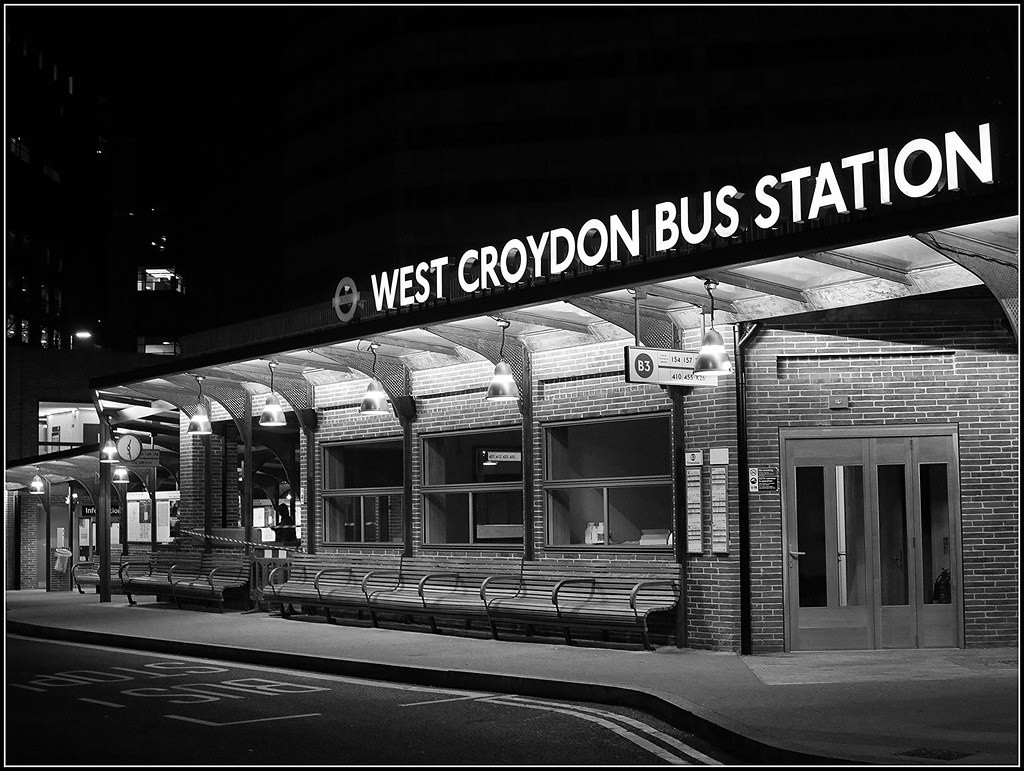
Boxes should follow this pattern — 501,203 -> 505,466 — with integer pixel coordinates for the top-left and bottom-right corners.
117,432 -> 143,461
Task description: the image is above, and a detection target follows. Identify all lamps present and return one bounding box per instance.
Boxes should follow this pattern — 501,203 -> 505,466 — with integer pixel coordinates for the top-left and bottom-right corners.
186,402 -> 213,435
99,438 -> 120,463
112,464 -> 130,483
692,327 -> 734,375
358,378 -> 390,416
259,394 -> 287,427
486,361 -> 520,401
29,475 -> 46,495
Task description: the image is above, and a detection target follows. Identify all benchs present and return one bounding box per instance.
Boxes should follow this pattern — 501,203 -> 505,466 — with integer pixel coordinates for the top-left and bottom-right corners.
262,551 -> 687,651
72,551 -> 252,614
